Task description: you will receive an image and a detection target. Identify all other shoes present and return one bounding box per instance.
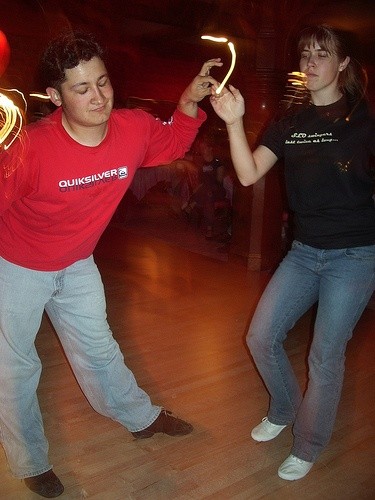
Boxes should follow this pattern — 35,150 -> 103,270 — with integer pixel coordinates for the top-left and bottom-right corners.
131,410 -> 193,439
23,469 -> 64,498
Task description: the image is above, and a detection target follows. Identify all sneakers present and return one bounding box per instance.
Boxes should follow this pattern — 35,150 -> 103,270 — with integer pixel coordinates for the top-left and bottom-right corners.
278,453 -> 315,481
251,417 -> 287,441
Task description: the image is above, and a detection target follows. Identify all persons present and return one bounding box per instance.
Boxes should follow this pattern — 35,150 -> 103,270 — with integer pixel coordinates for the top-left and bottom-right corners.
177,137 -> 232,236
210,25 -> 375,483
0,34 -> 222,498
